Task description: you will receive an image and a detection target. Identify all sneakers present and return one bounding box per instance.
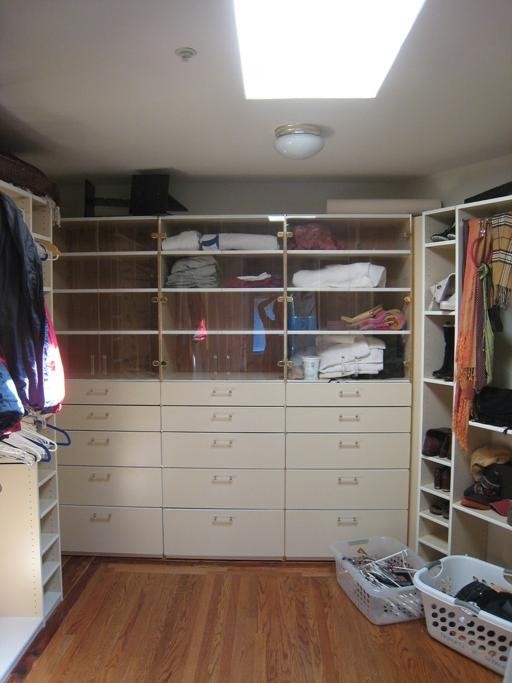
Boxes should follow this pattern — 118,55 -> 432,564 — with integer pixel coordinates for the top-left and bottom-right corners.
431,221 -> 456,242
433,469 -> 450,491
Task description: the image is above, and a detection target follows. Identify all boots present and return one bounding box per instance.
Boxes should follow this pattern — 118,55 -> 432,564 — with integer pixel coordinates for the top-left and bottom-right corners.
432,324 -> 454,382
422,429 -> 451,459
431,272 -> 455,311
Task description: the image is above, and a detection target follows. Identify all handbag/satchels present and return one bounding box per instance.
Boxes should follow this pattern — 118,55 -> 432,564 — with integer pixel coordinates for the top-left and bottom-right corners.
475,386 -> 512,435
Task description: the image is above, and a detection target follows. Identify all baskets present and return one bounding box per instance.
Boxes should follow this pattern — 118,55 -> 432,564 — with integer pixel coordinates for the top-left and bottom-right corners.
329,535 -> 512,678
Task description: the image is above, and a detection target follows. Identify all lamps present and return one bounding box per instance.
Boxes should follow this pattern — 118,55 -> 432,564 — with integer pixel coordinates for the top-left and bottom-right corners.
272,122 -> 328,162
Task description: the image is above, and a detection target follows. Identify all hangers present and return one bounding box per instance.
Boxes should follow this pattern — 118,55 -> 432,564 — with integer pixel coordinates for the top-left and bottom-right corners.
36,238 -> 61,262
0,417 -> 70,469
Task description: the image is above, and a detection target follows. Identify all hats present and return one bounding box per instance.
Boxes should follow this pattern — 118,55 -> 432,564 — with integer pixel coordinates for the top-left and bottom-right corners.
461,445 -> 512,527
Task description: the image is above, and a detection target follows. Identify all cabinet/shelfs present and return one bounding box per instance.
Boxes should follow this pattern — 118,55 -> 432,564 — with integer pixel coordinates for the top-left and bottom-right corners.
414,194 -> 512,578
52,212 -> 417,562
0,179 -> 65,682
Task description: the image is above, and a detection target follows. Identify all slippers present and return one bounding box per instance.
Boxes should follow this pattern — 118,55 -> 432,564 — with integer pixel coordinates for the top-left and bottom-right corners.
430,501 -> 449,519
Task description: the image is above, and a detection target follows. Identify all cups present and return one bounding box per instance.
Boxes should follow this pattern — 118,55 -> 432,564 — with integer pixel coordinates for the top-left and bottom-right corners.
302,355 -> 320,380
